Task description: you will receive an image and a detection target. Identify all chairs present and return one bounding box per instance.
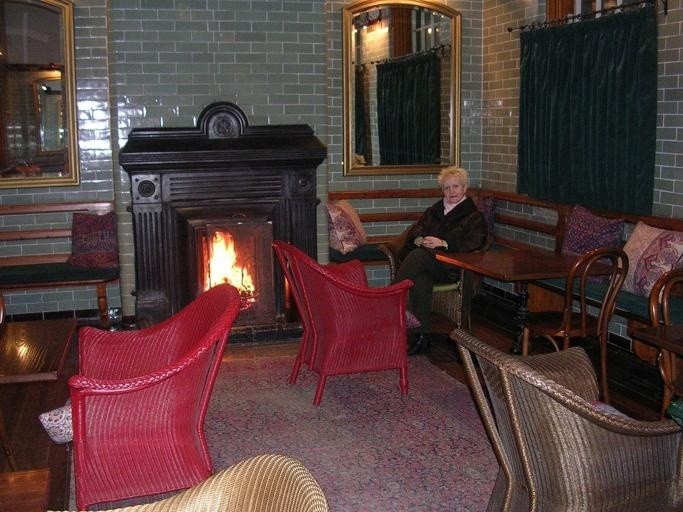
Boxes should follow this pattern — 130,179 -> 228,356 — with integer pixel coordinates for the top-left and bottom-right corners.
64,281 -> 238,511
520,245 -> 629,409
269,238 -> 415,404
649,267 -> 683,427
444,327 -> 682,512
45,453 -> 332,512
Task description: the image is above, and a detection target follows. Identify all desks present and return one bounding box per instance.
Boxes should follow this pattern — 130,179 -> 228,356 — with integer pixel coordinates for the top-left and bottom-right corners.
436,249 -> 625,353
0,313 -> 87,383
0,468 -> 54,512
626,321 -> 682,356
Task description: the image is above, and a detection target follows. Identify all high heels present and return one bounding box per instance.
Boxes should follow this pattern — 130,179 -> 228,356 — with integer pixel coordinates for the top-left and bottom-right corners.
407,334 -> 430,355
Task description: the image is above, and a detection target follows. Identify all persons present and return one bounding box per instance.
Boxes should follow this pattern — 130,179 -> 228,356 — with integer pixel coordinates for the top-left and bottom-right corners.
390,166 -> 486,354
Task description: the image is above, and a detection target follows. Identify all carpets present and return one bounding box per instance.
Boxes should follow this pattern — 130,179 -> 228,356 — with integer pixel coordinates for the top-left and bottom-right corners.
205,353 -> 498,511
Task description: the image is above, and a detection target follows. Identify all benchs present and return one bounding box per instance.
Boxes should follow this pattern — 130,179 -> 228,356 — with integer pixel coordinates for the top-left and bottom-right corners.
486,191 -> 682,339
0,202 -> 119,318
328,187 -> 486,268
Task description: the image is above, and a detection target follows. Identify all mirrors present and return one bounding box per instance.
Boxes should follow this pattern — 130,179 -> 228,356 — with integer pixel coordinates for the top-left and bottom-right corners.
1,0 -> 78,190
31,73 -> 65,158
340,3 -> 462,176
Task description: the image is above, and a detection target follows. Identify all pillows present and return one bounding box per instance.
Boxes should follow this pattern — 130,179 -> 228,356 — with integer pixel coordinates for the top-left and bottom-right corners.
65,208 -> 119,269
561,204 -> 626,269
607,217 -> 682,303
325,200 -> 369,255
